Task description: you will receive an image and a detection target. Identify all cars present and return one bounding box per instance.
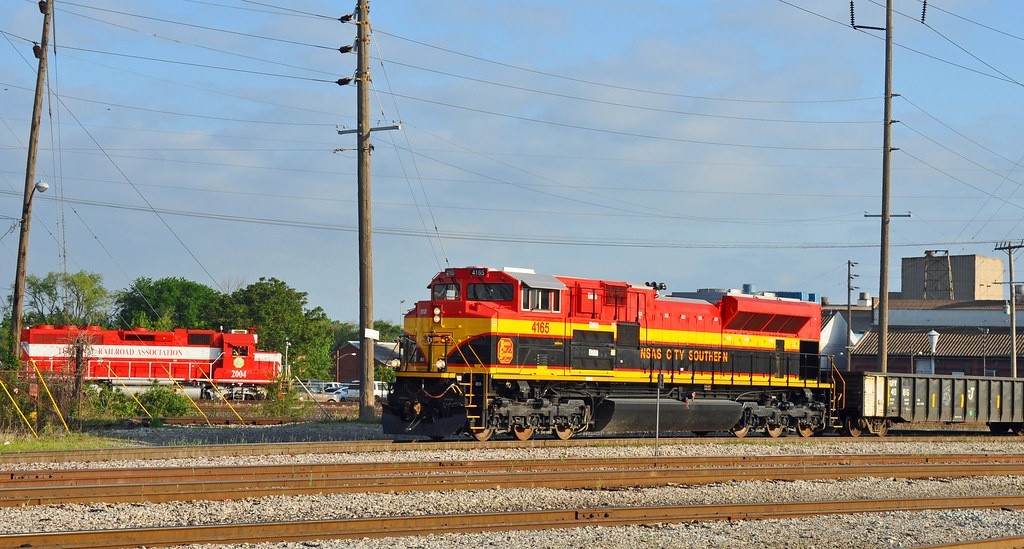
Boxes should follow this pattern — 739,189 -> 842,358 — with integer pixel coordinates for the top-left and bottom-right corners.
310,381 -> 360,393
322,386 -> 357,402
289,387 -> 325,401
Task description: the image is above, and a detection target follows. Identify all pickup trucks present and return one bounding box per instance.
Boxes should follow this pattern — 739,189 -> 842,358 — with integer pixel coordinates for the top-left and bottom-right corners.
346,380 -> 395,402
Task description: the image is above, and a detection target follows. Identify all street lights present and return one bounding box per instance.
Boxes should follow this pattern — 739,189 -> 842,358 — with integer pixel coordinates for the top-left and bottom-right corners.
9,180 -> 49,382
927,329 -> 942,375
336,349 -> 357,381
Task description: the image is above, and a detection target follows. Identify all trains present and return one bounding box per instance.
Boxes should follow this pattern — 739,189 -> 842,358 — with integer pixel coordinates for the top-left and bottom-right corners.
21,322 -> 284,404
379,266 -> 1024,439
